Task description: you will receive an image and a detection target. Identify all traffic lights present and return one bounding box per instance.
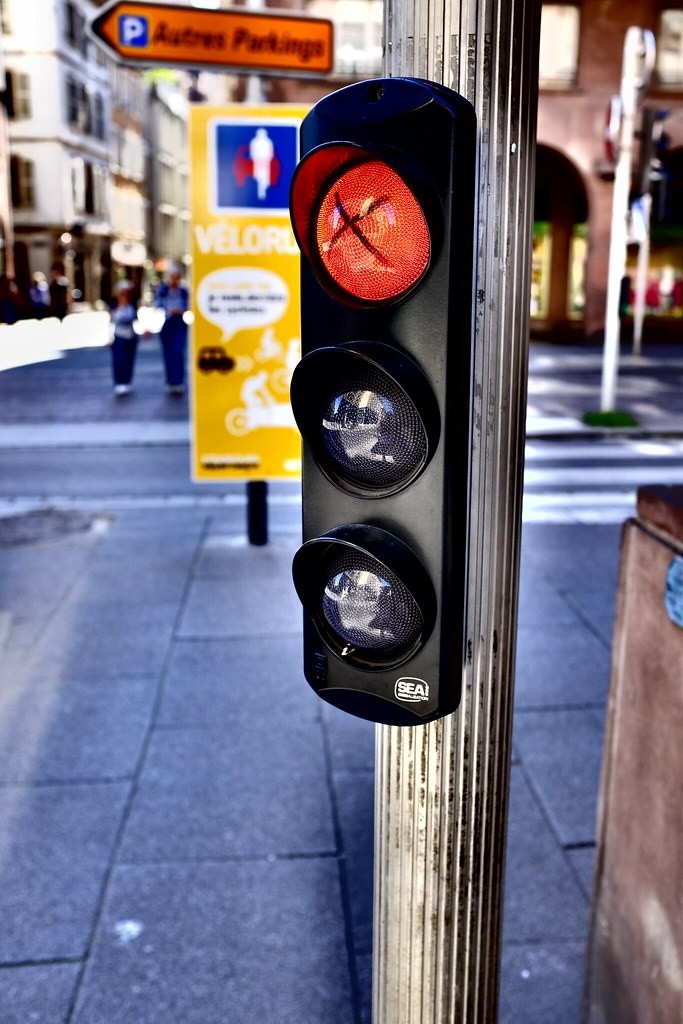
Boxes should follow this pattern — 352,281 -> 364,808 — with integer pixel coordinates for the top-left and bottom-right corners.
281,77 -> 480,728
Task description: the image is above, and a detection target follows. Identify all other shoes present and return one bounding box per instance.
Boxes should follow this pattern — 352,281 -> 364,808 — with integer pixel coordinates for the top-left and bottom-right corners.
114,383 -> 132,397
164,383 -> 183,397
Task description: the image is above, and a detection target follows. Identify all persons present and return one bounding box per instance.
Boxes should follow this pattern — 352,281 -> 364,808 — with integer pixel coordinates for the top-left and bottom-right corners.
29,262 -> 66,319
105,279 -> 141,397
143,260 -> 189,395
